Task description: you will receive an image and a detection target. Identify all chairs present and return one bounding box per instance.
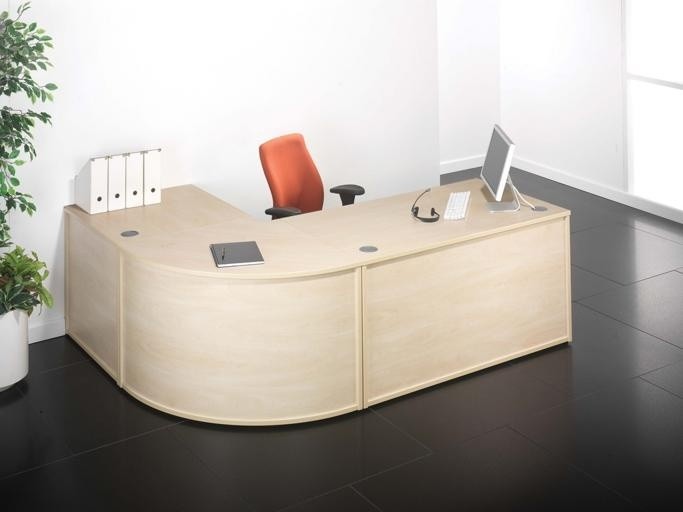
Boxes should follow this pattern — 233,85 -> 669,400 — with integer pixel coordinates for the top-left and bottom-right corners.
257,130 -> 366,219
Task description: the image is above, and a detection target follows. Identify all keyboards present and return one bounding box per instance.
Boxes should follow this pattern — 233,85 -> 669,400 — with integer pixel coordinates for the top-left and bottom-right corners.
444,190 -> 470,221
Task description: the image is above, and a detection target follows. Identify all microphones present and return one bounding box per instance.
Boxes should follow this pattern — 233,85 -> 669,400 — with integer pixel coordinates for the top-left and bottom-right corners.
414,188 -> 431,208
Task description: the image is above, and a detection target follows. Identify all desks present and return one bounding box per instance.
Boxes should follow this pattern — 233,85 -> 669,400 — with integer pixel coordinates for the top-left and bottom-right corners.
62,175 -> 572,427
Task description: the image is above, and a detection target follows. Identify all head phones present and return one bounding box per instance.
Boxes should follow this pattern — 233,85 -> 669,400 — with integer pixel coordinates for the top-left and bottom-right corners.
410,206 -> 441,223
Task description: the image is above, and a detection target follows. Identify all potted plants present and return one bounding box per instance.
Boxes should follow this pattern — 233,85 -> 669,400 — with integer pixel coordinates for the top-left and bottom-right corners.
1,0 -> 59,394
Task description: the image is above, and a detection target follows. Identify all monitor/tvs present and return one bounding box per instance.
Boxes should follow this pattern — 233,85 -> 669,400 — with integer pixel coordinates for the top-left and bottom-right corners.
479,124 -> 522,214
